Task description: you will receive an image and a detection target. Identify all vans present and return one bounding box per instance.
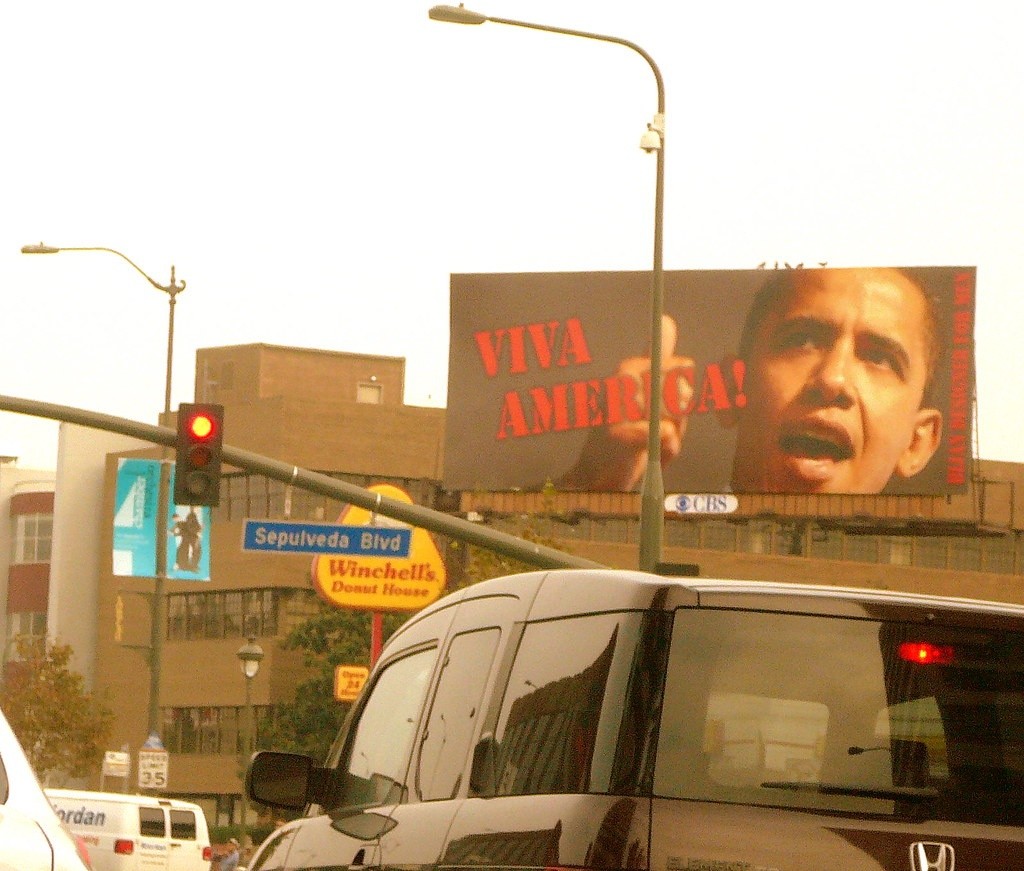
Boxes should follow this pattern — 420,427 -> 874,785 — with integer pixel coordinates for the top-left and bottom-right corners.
0,703 -> 92,870
43,788 -> 213,871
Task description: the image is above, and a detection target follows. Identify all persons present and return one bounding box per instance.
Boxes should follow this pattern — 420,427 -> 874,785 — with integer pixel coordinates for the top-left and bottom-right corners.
527,267 -> 945,493
218,838 -> 239,871
169,507 -> 204,572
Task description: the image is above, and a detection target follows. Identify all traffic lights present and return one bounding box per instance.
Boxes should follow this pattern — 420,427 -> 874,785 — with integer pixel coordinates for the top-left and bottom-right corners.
173,398 -> 226,508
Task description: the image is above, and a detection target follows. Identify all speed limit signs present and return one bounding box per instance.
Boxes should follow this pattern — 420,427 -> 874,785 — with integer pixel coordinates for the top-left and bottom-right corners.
137,749 -> 170,789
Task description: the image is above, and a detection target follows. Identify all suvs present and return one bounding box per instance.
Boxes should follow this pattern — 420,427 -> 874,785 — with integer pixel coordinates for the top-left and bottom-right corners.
239,566 -> 1023,870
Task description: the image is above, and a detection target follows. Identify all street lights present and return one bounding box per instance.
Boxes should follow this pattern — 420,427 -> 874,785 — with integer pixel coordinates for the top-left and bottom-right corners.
232,632 -> 268,845
425,5 -> 668,575
20,241 -> 186,795
